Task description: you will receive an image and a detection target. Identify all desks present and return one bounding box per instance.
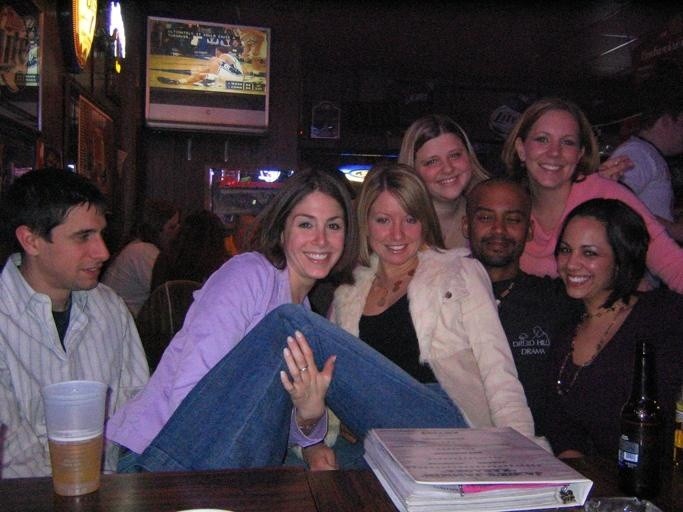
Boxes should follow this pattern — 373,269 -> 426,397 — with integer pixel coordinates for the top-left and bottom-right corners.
309,458 -> 628,512
1,465 -> 314,512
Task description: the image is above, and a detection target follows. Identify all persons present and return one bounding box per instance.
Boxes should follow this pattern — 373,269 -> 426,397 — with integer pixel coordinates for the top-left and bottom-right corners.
500,96 -> 683,295
103,162 -> 360,474
599,81 -> 683,244
397,113 -> 635,252
157,46 -> 244,86
544,198 -> 683,488
461,177 -> 586,457
100,196 -> 262,322
136,165 -> 536,474
0,167 -> 150,479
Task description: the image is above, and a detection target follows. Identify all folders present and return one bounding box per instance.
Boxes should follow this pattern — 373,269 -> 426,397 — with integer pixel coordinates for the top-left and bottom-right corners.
363,425 -> 593,512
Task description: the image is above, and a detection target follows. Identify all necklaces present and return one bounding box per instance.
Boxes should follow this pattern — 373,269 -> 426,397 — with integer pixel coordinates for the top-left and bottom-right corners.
376,266 -> 415,307
556,304 -> 626,397
496,270 -> 520,306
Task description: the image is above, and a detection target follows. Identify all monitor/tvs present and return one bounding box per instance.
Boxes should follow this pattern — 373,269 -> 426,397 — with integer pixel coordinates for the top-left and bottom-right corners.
143,13 -> 273,138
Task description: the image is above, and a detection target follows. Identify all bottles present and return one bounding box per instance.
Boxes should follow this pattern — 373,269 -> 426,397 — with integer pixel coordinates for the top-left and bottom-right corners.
617,336 -> 666,500
656,399 -> 683,512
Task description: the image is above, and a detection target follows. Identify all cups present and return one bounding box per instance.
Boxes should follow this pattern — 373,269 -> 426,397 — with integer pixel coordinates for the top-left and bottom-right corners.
40,381 -> 111,496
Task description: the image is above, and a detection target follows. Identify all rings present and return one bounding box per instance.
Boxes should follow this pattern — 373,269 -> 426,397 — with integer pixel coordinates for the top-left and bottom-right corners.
299,366 -> 308,371
612,158 -> 618,166
